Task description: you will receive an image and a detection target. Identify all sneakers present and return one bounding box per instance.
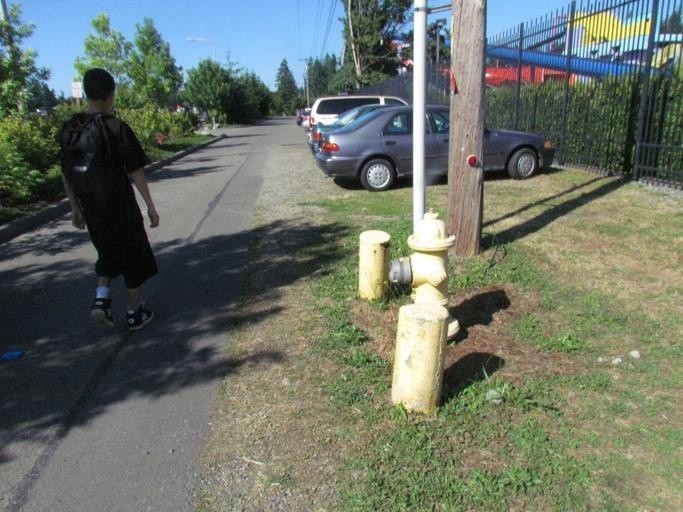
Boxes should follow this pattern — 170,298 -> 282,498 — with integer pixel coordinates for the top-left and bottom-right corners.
125,301 -> 154,331
90,297 -> 116,328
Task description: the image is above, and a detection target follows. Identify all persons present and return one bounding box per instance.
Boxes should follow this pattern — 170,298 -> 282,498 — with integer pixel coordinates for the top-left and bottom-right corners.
56,67 -> 161,333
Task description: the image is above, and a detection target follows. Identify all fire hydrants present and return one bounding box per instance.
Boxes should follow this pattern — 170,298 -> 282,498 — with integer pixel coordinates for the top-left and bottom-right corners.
389,208 -> 460,341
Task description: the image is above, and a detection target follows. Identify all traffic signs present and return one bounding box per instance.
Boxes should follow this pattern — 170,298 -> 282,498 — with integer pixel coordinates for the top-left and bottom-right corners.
186,37 -> 216,61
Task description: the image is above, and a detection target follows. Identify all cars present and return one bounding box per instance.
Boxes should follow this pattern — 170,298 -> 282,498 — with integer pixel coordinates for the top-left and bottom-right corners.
298,95 -> 555,191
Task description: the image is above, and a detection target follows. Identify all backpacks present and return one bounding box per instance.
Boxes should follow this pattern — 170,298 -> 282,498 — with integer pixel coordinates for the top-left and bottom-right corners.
61,109 -> 118,204
